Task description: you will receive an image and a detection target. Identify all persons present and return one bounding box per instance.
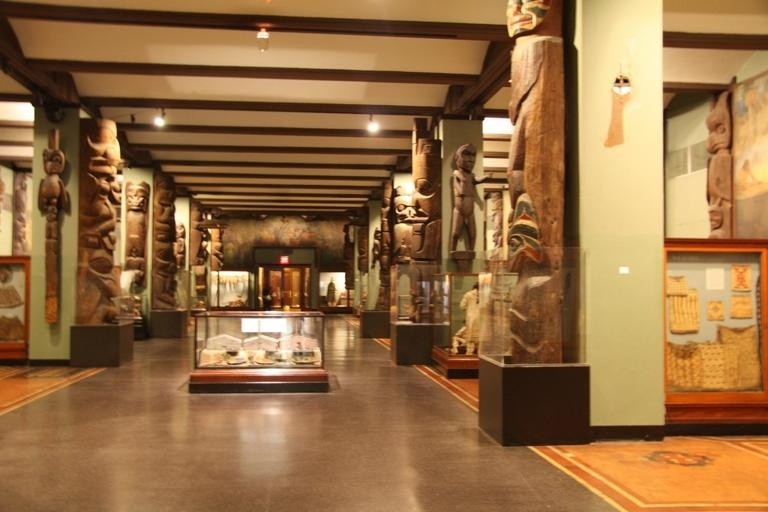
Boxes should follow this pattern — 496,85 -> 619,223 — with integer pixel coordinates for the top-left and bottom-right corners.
448,143 -> 486,250
